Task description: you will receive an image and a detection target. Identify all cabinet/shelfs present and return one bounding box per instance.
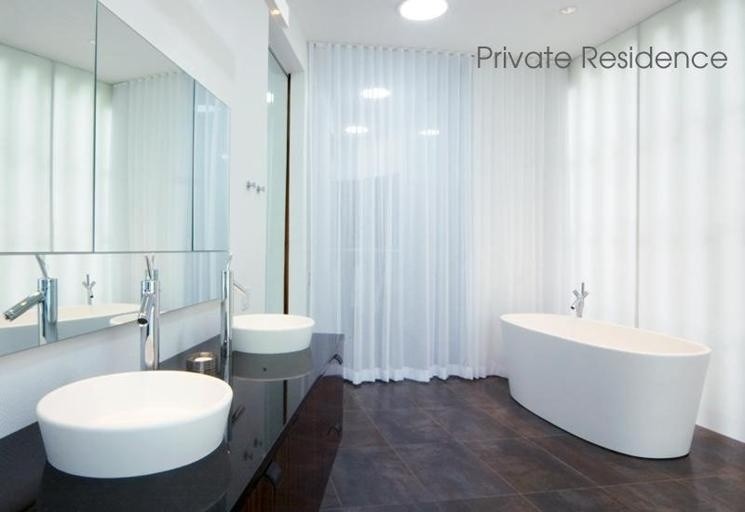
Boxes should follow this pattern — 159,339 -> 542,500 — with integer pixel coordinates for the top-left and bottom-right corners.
233,344 -> 342,512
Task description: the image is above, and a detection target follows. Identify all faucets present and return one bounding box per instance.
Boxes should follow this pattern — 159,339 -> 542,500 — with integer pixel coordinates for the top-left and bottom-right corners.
137,278 -> 160,371
3,255 -> 59,346
219,253 -> 248,360
570,282 -> 591,317
81,274 -> 97,305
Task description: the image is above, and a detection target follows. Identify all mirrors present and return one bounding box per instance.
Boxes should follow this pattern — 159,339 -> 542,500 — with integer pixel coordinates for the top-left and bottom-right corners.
1,2 -> 233,254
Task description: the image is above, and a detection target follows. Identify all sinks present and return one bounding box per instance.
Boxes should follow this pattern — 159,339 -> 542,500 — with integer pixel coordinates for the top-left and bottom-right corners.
107,310 -> 164,327
229,313 -> 316,355
34,369 -> 234,479
33,436 -> 234,512
229,348 -> 317,381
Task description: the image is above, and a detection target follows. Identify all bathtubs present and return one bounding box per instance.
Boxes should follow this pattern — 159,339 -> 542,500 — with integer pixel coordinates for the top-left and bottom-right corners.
499,312 -> 713,460
1,302 -> 142,355
